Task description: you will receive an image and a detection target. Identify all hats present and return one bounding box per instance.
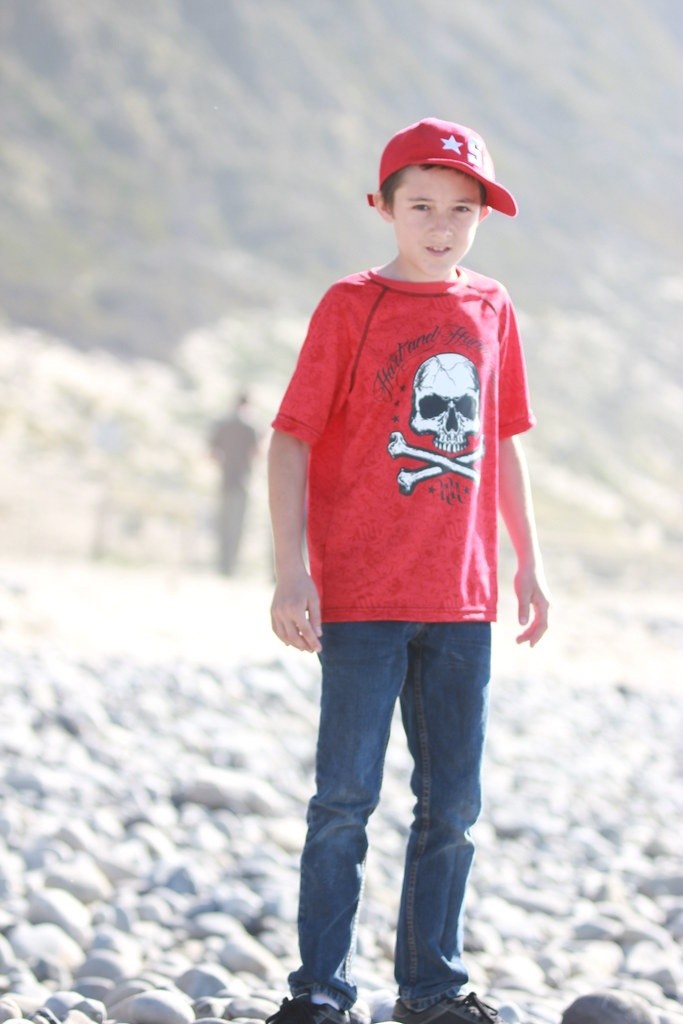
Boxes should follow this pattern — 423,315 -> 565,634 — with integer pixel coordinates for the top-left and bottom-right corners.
368,118 -> 517,217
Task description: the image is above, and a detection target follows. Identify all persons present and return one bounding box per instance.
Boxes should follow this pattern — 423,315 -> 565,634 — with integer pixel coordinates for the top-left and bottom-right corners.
268,115 -> 551,1024
211,389 -> 267,579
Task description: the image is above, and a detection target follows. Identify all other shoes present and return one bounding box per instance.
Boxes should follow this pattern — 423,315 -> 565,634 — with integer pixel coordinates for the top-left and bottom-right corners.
392,991 -> 508,1024
264,990 -> 350,1024
